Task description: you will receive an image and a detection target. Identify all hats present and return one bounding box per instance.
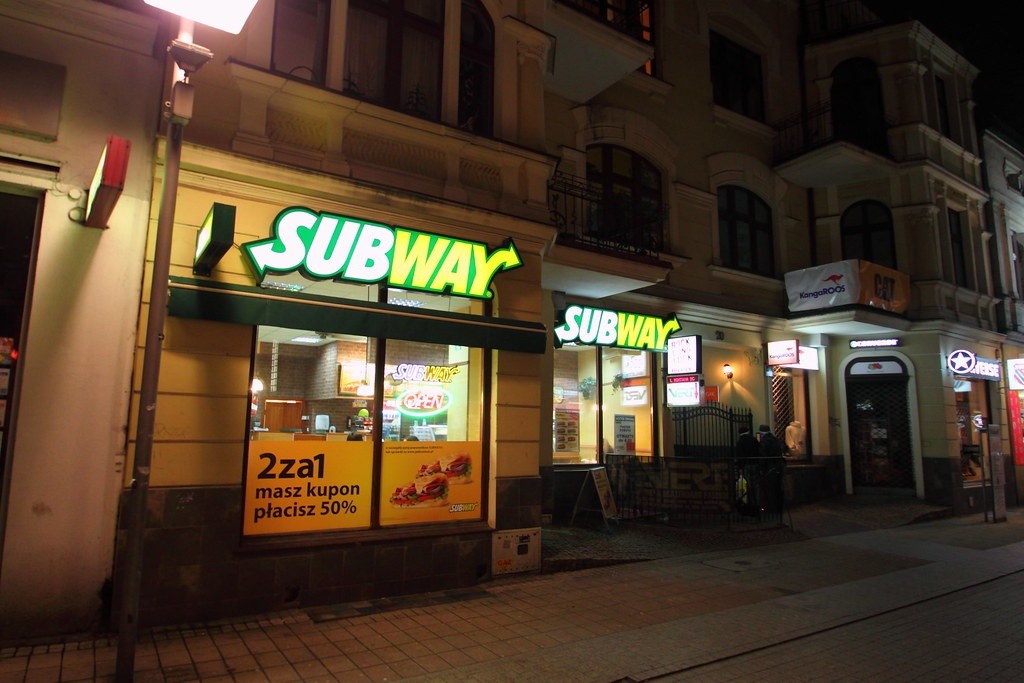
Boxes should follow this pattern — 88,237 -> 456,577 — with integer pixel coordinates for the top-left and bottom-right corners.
760,425 -> 770,432
739,427 -> 748,434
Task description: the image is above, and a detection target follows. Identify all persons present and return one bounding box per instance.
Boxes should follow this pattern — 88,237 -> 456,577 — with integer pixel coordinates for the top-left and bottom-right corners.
735,424 -> 784,501
347,432 -> 363,441
